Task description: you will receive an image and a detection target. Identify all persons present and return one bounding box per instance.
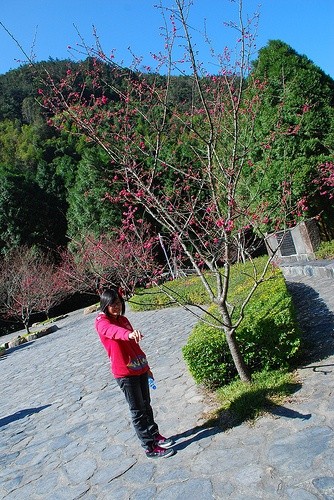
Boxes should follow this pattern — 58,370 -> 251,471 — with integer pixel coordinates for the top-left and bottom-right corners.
95,289 -> 175,459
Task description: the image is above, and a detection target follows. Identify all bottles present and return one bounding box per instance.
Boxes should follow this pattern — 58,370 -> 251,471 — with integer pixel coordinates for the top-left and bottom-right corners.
147,376 -> 156,389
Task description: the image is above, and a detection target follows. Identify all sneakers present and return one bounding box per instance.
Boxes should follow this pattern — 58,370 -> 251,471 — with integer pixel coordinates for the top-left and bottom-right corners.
154,433 -> 175,447
145,445 -> 175,460
